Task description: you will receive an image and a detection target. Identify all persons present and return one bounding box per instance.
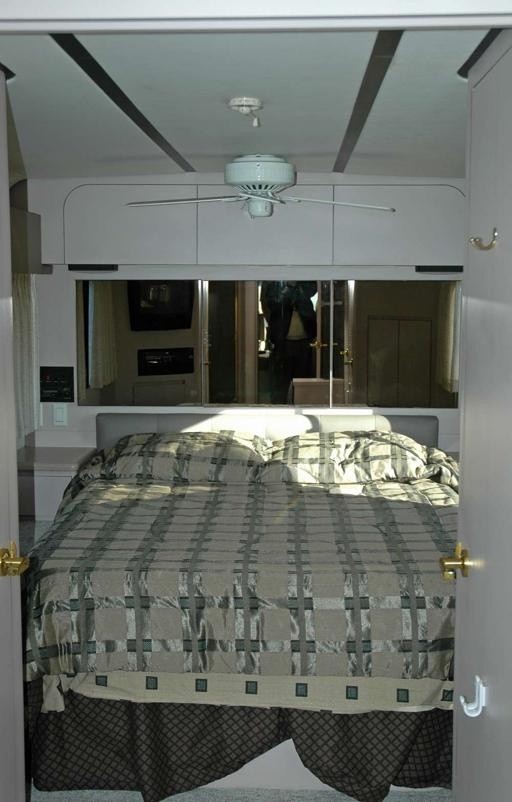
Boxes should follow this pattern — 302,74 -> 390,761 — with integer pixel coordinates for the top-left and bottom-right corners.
260,280 -> 317,403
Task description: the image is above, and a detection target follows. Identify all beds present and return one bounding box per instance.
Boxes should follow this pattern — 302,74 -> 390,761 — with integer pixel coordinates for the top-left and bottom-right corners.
21,410 -> 460,802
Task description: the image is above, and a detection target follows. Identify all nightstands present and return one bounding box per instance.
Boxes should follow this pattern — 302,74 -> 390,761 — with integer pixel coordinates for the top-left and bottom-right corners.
17,445 -> 96,522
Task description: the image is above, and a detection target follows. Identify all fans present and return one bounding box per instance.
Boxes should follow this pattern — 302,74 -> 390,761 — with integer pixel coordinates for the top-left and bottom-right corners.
122,154 -> 396,218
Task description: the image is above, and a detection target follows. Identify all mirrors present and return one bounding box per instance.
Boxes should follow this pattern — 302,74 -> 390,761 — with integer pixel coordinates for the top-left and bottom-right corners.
75,277 -> 463,410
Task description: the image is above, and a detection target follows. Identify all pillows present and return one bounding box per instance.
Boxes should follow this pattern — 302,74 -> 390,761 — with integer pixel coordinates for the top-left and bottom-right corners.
258,429 -> 437,485
100,431 -> 270,484
